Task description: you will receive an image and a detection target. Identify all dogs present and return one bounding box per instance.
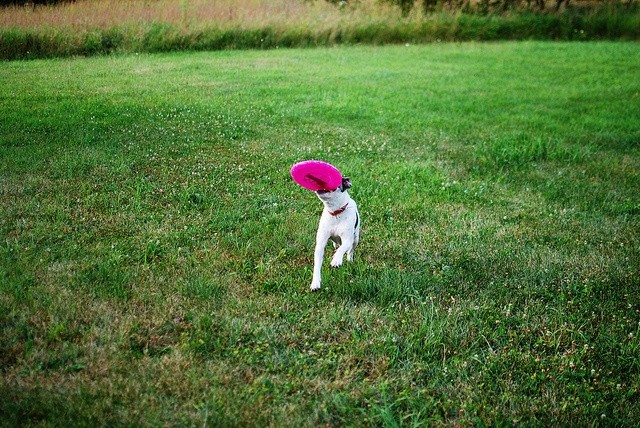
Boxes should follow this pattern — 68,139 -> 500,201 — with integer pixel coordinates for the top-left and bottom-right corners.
309,176 -> 361,293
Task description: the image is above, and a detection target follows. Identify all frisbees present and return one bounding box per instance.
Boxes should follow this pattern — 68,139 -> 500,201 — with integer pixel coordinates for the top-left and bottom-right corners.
290,160 -> 342,191
